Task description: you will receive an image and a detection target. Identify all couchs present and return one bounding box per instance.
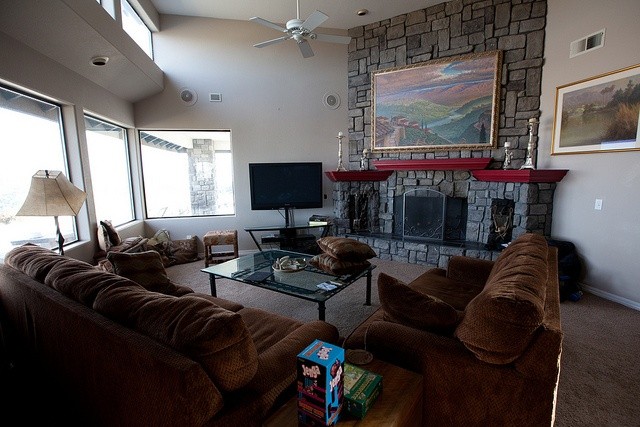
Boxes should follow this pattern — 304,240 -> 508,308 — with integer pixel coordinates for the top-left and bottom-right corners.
0,240 -> 341,427
341,232 -> 564,426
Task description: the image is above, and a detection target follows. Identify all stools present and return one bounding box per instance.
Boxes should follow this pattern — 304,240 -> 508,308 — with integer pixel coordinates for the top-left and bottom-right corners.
204,229 -> 239,269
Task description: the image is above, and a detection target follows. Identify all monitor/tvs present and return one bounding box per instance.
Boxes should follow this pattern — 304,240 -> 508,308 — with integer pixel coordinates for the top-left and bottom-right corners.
249,162 -> 322,225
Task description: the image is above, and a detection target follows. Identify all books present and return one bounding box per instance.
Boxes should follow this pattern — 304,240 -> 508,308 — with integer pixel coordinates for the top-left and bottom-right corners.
309,214 -> 330,221
309,222 -> 327,225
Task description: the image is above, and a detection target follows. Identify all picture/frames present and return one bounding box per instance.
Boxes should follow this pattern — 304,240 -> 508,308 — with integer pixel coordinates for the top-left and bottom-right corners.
370,49 -> 503,154
550,63 -> 640,156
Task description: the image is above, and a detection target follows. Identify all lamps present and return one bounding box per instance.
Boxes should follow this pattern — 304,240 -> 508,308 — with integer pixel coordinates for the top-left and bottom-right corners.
19,168 -> 87,259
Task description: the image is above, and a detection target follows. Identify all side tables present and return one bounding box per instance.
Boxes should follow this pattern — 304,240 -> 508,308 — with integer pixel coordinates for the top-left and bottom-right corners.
260,346 -> 425,426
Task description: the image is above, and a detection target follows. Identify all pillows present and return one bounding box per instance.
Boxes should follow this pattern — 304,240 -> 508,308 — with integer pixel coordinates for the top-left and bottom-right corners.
377,269 -> 467,339
307,253 -> 372,277
96,220 -> 123,252
315,236 -> 378,261
106,250 -> 180,295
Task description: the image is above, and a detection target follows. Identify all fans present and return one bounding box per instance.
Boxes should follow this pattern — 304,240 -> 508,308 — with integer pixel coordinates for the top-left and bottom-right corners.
246,0 -> 352,59
